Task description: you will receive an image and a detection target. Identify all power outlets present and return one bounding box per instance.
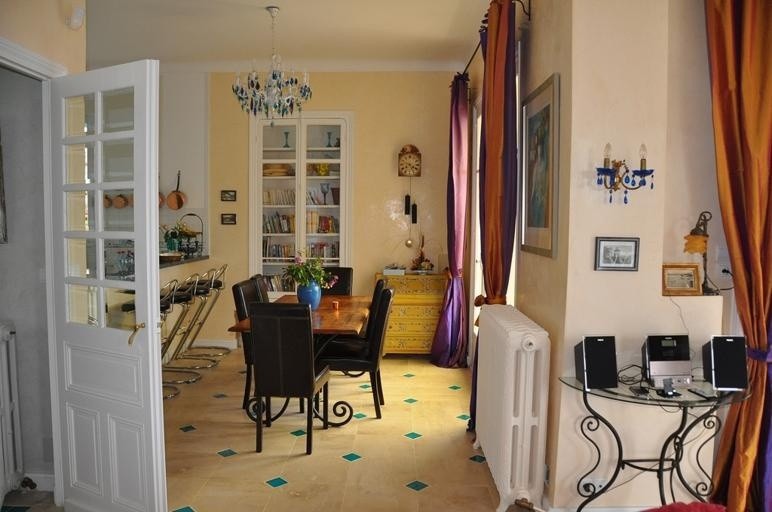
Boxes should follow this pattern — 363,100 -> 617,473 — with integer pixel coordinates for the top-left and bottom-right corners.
718,263 -> 734,281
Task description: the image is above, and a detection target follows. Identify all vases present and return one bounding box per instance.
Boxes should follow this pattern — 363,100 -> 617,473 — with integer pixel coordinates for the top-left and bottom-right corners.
297,279 -> 322,312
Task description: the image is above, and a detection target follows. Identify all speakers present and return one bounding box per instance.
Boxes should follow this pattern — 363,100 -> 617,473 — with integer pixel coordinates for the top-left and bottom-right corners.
575,336 -> 618,391
702,336 -> 749,393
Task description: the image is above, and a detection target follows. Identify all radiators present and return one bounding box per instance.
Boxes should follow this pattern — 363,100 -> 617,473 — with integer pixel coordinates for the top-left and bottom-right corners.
0,315 -> 28,502
473,304 -> 554,512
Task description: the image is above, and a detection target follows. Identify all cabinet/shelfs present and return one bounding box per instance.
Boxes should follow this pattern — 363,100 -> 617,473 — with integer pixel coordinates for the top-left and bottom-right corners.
376,272 -> 451,359
247,110 -> 356,303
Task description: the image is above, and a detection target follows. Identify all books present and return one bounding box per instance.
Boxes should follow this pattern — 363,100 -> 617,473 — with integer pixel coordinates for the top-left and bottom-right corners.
262,188 -> 339,293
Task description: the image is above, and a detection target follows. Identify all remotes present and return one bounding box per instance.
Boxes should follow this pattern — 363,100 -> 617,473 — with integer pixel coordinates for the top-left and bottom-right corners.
689,388 -> 716,400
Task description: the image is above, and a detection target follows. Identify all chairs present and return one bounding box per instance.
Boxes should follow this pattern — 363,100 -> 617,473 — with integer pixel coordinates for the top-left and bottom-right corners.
247,301 -> 333,457
316,286 -> 396,424
231,280 -> 306,414
249,273 -> 269,308
322,264 -> 355,298
331,279 -> 388,379
115,262 -> 233,402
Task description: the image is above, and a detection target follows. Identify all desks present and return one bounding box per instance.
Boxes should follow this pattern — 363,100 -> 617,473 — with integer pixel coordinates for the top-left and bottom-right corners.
558,374 -> 757,512
228,293 -> 375,428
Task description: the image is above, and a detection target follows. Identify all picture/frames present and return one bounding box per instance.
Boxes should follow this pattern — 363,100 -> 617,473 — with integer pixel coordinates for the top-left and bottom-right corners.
594,236 -> 641,273
662,263 -> 703,298
221,190 -> 236,202
520,69 -> 562,262
221,214 -> 237,225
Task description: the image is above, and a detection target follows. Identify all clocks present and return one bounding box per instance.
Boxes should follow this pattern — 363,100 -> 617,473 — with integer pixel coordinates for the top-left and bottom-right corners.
397,143 -> 423,250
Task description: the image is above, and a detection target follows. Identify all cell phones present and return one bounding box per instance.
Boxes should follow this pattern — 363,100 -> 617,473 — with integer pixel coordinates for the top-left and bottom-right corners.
629,386 -> 648,396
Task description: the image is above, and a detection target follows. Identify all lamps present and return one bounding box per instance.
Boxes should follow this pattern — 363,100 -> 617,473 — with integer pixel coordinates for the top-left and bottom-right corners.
683,206 -> 721,295
229,7 -> 313,120
594,141 -> 657,207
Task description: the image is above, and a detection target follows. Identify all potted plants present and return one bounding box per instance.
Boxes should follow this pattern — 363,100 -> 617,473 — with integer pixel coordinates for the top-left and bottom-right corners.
158,221 -> 195,265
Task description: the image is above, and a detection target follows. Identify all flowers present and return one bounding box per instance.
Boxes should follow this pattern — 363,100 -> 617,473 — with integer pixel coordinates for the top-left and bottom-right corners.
283,255 -> 341,292
